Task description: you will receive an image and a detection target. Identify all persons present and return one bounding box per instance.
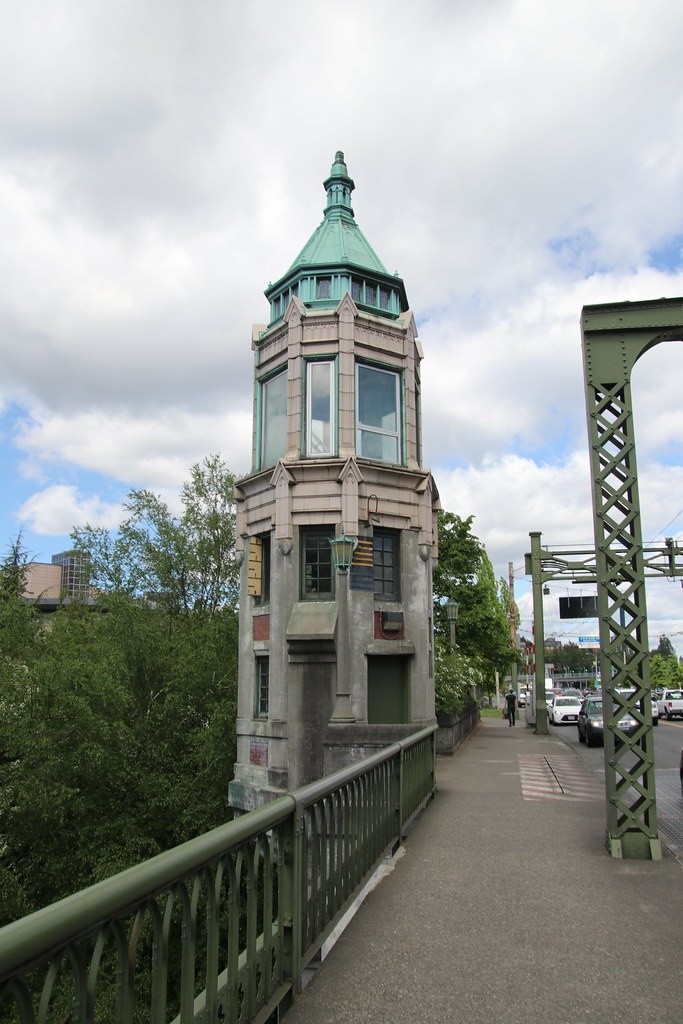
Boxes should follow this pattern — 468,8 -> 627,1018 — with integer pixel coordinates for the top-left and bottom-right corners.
504,690 -> 518,726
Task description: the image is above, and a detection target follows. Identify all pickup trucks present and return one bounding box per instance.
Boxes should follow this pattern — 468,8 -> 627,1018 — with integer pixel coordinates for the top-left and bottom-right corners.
656,690 -> 683,721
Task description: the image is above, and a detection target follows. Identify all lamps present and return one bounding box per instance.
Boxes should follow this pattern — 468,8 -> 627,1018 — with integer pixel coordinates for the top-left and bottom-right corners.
328,524 -> 357,575
364,493 -> 380,527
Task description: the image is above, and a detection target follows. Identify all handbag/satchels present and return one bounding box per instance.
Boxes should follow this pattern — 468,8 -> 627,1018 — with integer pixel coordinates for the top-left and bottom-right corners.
502,709 -> 509,719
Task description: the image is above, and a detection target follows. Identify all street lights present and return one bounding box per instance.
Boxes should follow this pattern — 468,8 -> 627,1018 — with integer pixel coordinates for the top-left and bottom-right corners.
445,598 -> 459,647
328,525 -> 356,723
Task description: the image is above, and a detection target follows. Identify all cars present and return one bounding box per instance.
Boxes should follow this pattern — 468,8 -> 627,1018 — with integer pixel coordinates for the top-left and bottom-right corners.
615,689 -> 659,727
656,689 -> 662,695
577,697 -> 641,748
583,688 -> 598,697
551,688 -> 564,697
548,697 -> 582,726
562,689 -> 585,702
545,692 -> 556,713
518,687 -> 530,708
